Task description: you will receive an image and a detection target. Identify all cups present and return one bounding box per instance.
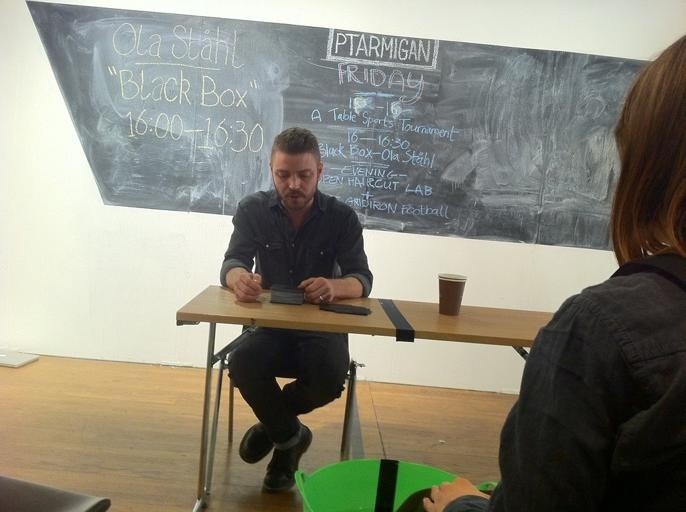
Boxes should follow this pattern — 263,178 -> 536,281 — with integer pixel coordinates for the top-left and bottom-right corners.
437,273 -> 467,316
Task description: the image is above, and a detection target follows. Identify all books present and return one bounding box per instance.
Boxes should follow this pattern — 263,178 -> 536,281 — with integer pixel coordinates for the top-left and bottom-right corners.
268,283 -> 305,305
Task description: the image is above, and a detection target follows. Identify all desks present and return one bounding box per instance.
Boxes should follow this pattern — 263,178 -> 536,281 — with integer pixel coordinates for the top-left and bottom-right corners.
174,283 -> 562,512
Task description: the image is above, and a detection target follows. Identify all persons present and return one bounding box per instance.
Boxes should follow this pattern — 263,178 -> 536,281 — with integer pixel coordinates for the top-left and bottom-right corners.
218,124 -> 374,497
413,32 -> 685,512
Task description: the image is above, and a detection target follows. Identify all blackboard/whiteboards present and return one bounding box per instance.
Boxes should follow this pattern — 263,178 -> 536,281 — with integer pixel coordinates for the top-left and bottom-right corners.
26,1 -> 655,252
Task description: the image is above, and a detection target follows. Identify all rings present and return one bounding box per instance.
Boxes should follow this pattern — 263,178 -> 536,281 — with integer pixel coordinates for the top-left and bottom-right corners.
319,296 -> 324,302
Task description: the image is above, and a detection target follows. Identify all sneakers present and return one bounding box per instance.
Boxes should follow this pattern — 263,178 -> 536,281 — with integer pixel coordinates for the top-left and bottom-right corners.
237,424 -> 270,464
264,423 -> 313,491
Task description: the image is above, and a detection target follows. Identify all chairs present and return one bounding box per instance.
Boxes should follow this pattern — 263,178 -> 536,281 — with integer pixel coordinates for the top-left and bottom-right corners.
205,326 -> 360,504
